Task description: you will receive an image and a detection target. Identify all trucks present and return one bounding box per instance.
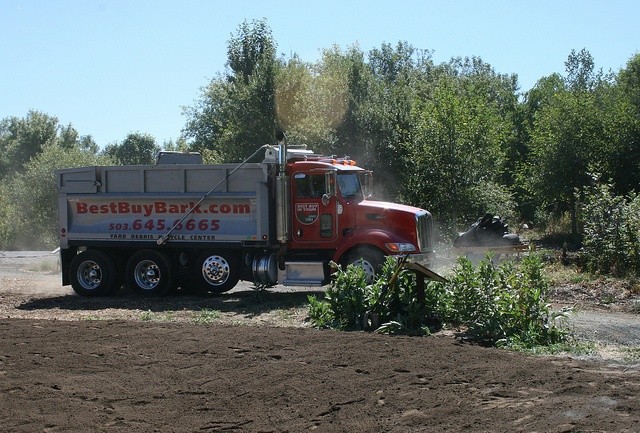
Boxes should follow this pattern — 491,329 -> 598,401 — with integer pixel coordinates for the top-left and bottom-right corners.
55,129 -> 433,297
